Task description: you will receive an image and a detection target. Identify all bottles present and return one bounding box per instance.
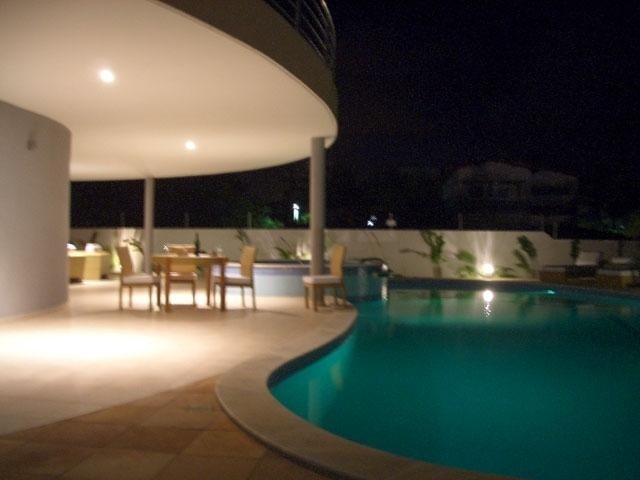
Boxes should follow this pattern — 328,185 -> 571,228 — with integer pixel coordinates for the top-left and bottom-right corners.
194,232 -> 201,257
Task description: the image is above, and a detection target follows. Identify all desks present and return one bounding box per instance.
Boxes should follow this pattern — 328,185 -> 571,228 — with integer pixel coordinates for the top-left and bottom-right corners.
149,253 -> 228,312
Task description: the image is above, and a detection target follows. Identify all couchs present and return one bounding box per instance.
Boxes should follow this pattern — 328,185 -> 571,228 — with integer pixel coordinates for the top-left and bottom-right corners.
67,251 -> 112,282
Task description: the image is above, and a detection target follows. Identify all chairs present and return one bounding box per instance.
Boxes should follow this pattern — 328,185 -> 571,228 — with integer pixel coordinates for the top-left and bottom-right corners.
537,248 -> 639,291
303,244 -> 347,312
115,247 -> 160,313
213,246 -> 258,311
165,244 -> 198,308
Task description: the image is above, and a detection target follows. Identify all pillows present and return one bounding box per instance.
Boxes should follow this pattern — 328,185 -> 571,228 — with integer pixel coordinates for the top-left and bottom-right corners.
67,243 -> 78,251
84,243 -> 104,250
